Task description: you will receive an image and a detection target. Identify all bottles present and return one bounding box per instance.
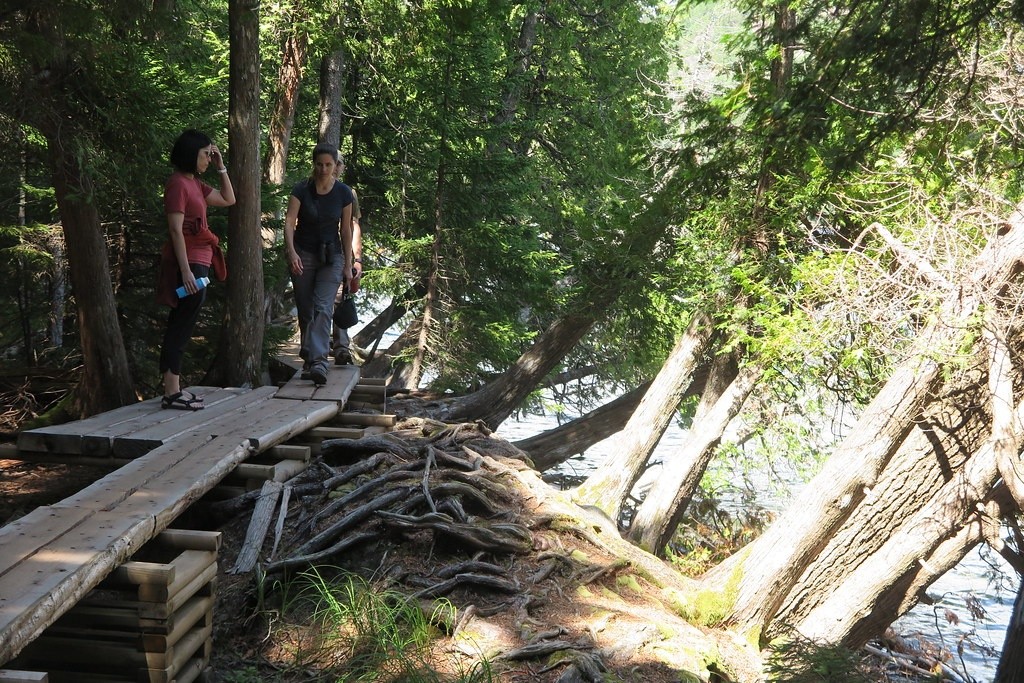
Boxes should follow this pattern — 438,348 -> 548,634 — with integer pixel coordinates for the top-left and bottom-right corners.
176,277 -> 210,298
350,269 -> 358,293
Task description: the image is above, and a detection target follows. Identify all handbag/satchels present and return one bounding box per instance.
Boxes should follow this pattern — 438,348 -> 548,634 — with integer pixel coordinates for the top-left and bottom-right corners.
333,298 -> 359,329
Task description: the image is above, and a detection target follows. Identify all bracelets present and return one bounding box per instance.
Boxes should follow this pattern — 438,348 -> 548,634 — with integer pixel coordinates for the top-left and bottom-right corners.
216,169 -> 227,174
354,259 -> 362,263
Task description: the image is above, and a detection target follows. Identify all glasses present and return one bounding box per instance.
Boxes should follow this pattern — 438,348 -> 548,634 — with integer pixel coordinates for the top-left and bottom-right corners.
199,150 -> 213,157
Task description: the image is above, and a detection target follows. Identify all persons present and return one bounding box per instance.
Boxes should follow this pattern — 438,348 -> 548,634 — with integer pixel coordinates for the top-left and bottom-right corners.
160,128 -> 236,410
284,142 -> 355,384
327,149 -> 362,366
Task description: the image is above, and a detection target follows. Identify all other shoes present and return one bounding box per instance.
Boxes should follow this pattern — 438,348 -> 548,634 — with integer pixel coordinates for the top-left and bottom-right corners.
310,368 -> 327,385
335,352 -> 350,365
301,368 -> 311,380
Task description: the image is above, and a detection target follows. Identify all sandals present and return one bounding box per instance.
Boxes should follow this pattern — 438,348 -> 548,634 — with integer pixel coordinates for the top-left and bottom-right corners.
180,389 -> 203,402
161,393 -> 204,410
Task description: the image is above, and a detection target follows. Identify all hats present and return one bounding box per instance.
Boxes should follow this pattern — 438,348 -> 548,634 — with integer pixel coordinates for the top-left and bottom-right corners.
337,150 -> 343,164
